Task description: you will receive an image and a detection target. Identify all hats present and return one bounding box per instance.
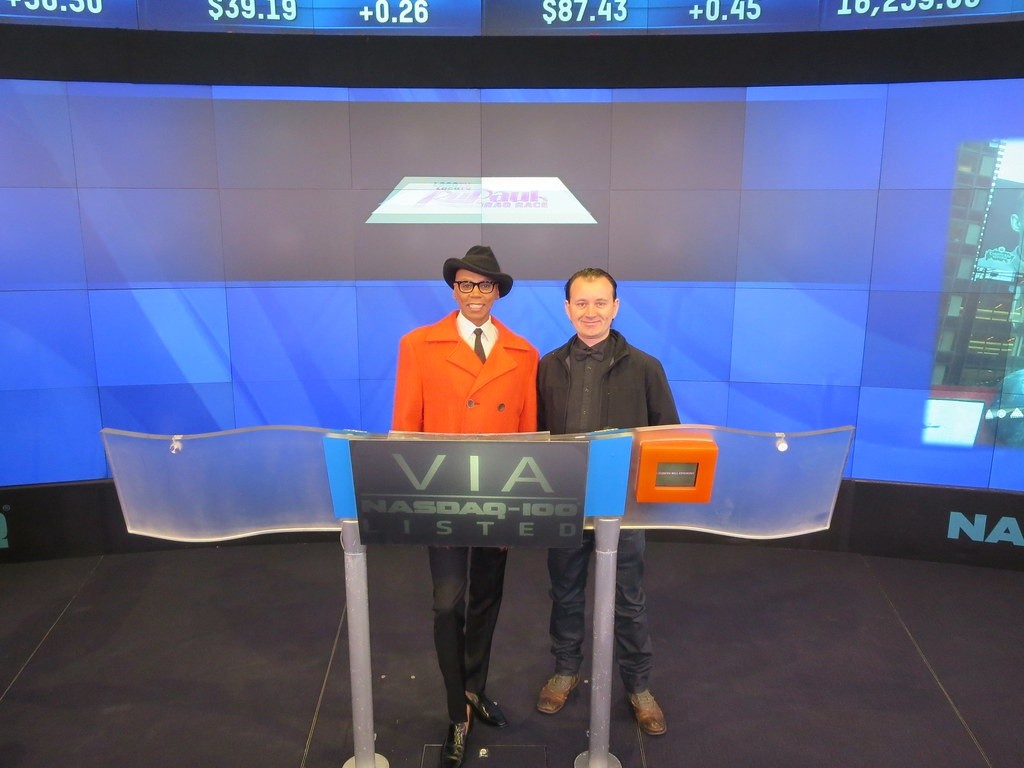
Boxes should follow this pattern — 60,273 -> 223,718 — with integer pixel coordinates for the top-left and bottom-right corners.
442,245 -> 513,298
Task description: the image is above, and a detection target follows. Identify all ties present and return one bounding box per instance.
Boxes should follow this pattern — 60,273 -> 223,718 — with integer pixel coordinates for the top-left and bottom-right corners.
473,328 -> 486,365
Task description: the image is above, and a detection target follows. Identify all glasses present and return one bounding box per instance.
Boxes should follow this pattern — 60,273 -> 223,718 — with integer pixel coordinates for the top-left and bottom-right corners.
454,280 -> 500,294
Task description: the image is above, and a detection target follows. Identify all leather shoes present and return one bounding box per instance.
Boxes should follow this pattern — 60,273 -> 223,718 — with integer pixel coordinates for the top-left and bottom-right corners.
440,703 -> 473,768
465,690 -> 508,729
624,687 -> 666,735
537,670 -> 580,714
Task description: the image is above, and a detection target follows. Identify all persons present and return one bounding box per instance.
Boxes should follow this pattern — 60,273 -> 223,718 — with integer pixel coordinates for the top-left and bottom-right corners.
532,267 -> 668,736
978,190 -> 1023,278
390,246 -> 541,768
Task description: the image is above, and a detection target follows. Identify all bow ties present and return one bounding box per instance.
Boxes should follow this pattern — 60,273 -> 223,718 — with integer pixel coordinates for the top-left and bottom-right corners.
574,346 -> 605,362
